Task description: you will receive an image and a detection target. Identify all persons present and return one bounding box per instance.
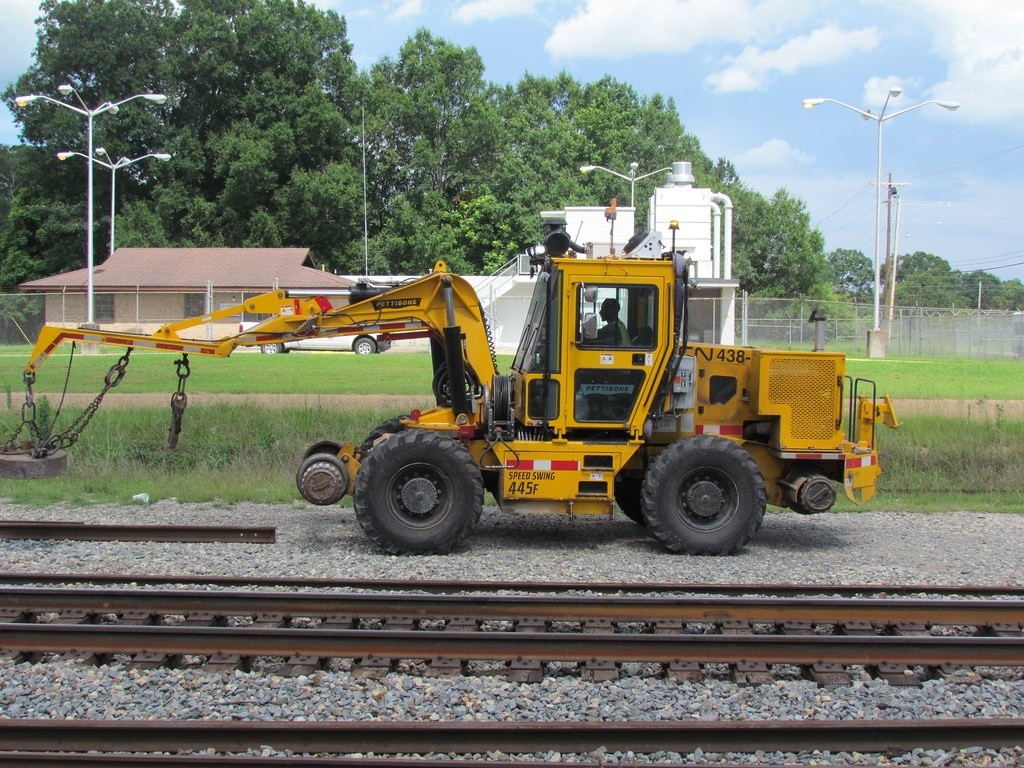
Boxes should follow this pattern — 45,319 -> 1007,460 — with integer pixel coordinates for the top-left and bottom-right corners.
583,298 -> 634,420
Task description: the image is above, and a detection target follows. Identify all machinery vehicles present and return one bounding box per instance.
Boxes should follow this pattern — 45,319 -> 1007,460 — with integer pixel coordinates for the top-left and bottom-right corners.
1,217 -> 900,553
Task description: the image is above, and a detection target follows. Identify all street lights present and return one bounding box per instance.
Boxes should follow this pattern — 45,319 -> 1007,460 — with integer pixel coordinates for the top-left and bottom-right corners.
14,84 -> 167,323
804,87 -> 960,331
579,162 -> 672,207
56,146 -> 173,258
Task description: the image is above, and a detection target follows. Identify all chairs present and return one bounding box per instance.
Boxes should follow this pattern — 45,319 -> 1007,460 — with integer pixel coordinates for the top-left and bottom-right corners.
637,327 -> 653,346
